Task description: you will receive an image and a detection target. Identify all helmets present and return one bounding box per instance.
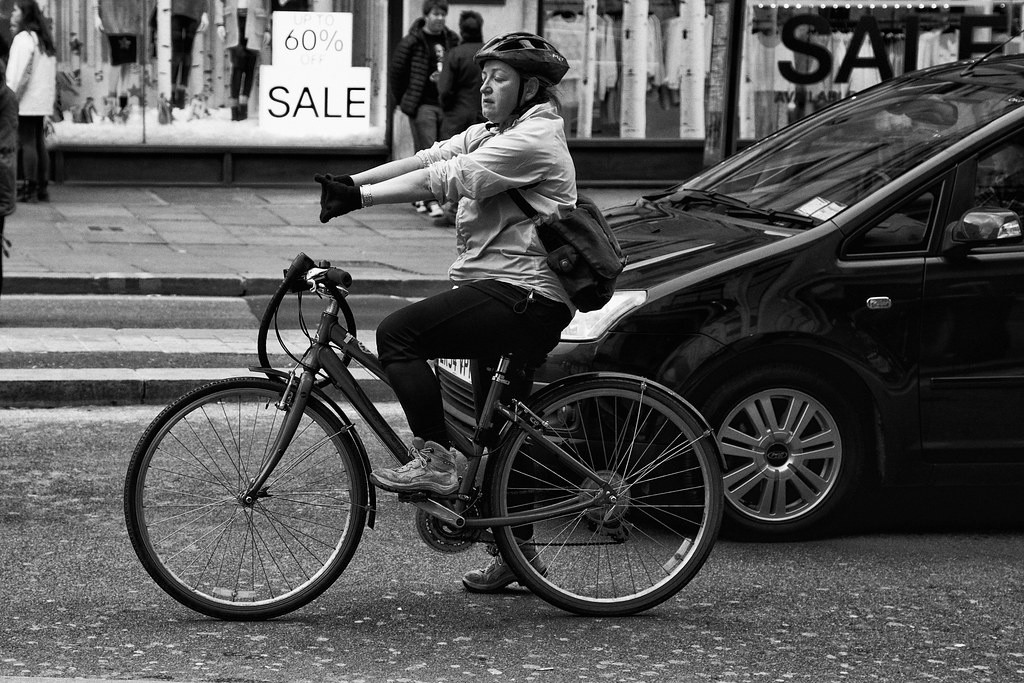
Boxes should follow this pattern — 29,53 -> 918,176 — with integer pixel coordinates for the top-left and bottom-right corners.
472,32 -> 570,84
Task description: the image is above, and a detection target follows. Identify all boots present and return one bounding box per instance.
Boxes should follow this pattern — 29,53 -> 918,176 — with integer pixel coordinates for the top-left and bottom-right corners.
38,181 -> 49,200
16,178 -> 38,202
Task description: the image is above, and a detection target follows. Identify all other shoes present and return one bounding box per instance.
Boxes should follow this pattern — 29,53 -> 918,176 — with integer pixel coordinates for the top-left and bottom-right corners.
427,201 -> 443,216
415,201 -> 427,212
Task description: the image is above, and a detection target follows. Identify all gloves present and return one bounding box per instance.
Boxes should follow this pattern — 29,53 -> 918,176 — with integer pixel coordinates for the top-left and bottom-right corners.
314,173 -> 362,223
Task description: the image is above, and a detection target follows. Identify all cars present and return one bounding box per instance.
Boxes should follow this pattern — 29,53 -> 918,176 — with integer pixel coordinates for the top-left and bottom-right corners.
430,54 -> 1024,543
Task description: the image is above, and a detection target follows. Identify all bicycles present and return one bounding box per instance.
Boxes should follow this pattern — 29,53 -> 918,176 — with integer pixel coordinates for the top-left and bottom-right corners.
121,250 -> 728,618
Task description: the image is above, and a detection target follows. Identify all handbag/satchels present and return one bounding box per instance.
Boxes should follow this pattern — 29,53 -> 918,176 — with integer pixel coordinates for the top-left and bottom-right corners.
536,192 -> 627,313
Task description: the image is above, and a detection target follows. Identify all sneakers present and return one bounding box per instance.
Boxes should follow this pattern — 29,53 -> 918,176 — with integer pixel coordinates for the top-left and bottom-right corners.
370,437 -> 459,495
462,537 -> 548,591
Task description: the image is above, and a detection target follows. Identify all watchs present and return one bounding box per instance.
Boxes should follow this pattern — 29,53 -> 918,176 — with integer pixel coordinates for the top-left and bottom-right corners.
362,183 -> 376,210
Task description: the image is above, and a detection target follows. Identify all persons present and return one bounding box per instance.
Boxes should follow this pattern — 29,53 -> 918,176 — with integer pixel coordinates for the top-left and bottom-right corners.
392,0 -> 491,219
0,0 -> 59,288
92,0 -> 306,122
314,31 -> 629,592
